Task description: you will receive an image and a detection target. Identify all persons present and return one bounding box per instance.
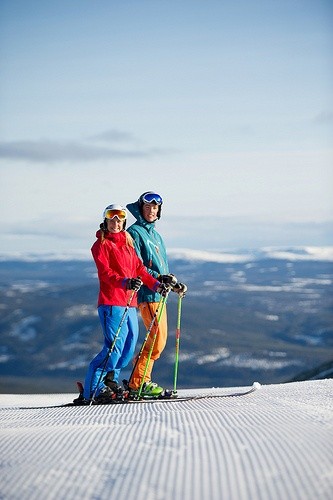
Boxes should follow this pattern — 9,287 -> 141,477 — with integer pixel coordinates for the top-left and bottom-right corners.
83,204 -> 170,402
125,191 -> 188,397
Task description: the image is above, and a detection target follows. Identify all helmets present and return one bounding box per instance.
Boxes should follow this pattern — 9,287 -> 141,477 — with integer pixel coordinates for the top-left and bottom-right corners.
104,204 -> 126,220
139,192 -> 160,207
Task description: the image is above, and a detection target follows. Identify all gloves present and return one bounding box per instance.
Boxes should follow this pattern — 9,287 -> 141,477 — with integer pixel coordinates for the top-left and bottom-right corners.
157,283 -> 173,298
172,281 -> 187,298
127,278 -> 144,292
160,274 -> 178,287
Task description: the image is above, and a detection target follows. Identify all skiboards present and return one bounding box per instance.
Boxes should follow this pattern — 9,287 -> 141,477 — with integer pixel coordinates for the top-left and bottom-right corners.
18,380 -> 261,409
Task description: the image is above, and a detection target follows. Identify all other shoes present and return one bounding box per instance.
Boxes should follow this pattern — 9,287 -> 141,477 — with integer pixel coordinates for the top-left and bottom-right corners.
107,381 -> 121,393
131,381 -> 163,394
99,388 -> 114,397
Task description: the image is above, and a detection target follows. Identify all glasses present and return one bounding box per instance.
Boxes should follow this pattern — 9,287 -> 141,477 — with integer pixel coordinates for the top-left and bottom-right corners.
105,209 -> 127,221
143,194 -> 162,206
